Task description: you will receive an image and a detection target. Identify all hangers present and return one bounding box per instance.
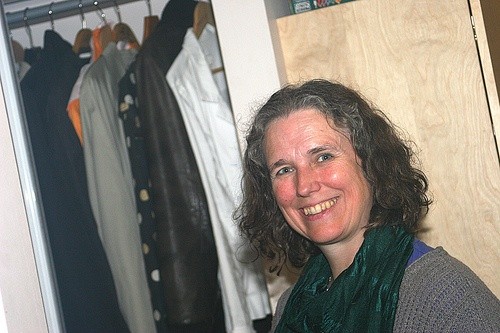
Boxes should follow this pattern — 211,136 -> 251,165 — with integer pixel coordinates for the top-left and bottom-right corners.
11,0 -> 211,72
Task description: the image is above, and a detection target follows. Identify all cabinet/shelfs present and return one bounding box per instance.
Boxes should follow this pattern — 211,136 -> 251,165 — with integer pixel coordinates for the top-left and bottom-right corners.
276,0 -> 500,333
0,0 -> 274,333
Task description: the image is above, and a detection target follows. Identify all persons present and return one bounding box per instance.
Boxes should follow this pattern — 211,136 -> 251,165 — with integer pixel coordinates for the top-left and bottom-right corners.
238,79 -> 500,333
